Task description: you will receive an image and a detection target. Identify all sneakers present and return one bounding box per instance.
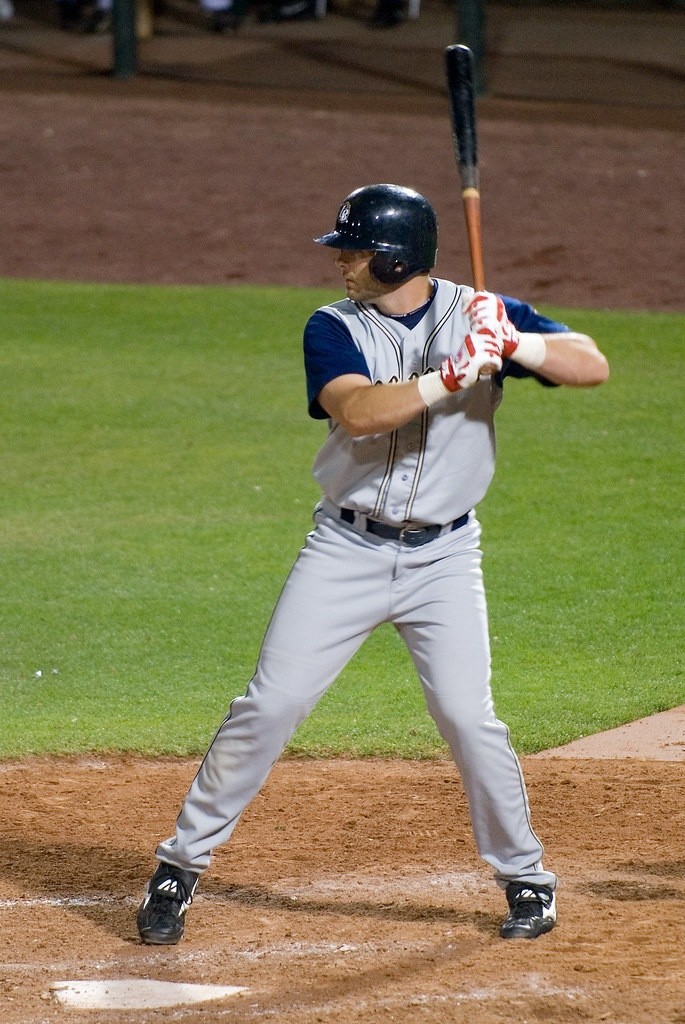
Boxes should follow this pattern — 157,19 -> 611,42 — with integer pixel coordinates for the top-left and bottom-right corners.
499,878 -> 557,939
136,861 -> 200,945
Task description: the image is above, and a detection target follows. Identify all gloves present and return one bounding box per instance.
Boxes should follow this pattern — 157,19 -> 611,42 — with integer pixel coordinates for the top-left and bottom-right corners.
462,290 -> 519,357
438,325 -> 504,393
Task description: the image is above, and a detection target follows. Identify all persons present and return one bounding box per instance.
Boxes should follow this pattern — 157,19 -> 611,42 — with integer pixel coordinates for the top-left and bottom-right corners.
136,184 -> 612,943
0,0 -> 409,30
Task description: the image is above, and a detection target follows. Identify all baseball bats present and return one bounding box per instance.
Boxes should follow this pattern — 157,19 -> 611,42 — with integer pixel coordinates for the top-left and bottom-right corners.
443,43 -> 497,376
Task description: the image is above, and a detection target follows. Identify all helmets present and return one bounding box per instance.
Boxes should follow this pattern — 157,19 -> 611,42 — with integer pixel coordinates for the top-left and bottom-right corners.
313,184 -> 439,288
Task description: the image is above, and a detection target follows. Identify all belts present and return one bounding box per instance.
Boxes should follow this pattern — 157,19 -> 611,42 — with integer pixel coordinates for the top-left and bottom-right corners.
340,508 -> 471,548
